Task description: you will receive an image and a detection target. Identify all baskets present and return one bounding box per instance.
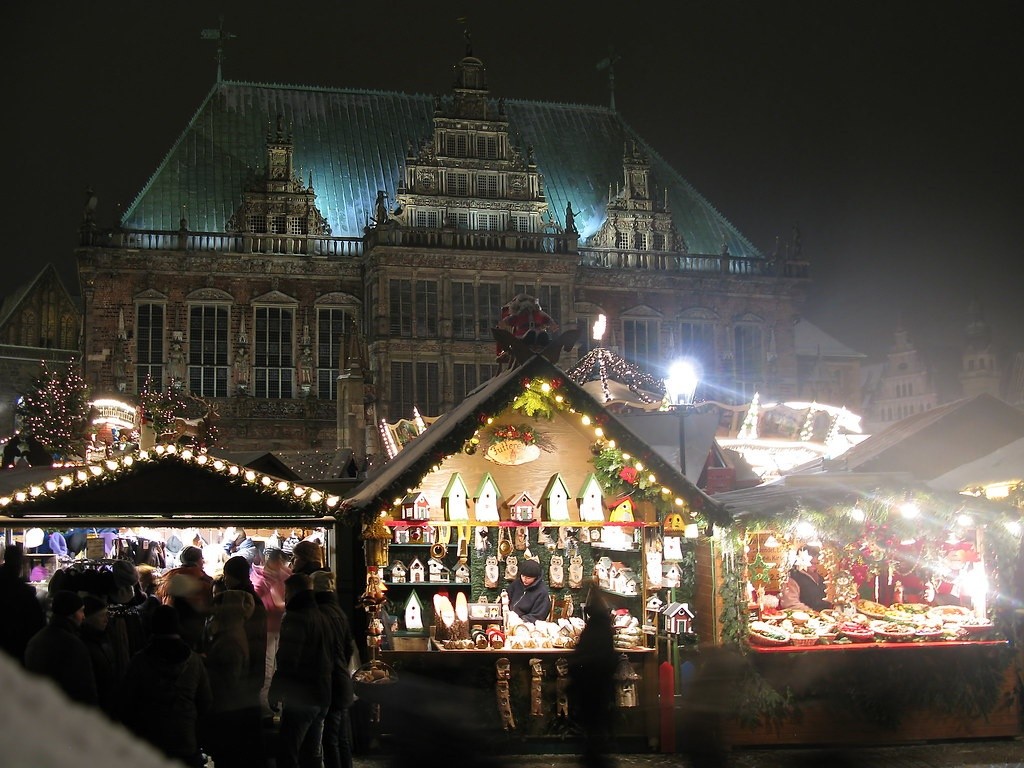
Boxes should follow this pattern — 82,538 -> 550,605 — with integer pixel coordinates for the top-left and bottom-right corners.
351,659 -> 399,704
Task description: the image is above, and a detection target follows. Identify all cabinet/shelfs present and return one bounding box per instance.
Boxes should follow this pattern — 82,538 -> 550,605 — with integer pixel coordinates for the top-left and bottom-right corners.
368,522 -> 661,647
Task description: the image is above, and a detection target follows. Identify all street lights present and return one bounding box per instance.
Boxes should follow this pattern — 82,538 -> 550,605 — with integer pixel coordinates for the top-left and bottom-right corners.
664,353 -> 694,479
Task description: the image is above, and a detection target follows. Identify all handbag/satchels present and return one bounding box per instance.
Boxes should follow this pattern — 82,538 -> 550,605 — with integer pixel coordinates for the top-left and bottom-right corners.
48,561 -> 146,606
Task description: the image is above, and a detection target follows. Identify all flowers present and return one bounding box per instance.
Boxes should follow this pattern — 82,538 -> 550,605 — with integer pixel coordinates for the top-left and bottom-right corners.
482,424 -> 558,455
586,448 -> 664,504
741,502 -> 982,605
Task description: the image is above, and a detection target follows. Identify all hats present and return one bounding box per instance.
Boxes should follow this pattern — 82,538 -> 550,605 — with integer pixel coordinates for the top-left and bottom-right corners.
82,596 -> 109,623
112,559 -> 140,590
180,547 -> 203,564
520,560 -> 541,577
292,541 -> 322,568
51,589 -> 84,616
309,570 -> 336,593
144,605 -> 186,635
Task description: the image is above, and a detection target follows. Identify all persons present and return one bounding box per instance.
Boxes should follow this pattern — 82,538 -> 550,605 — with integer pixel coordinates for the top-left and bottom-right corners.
31,557 -> 53,582
249,541 -> 355,768
566,588 -> 625,768
566,202 -> 581,232
502,560 -> 552,622
0,545 -> 47,667
793,222 -> 800,257
780,545 -> 829,610
681,645 -> 740,768
375,191 -> 389,224
24,527 -> 275,768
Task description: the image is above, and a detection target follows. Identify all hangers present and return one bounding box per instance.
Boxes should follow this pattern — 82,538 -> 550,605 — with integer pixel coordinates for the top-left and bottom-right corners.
57,559 -> 113,573
